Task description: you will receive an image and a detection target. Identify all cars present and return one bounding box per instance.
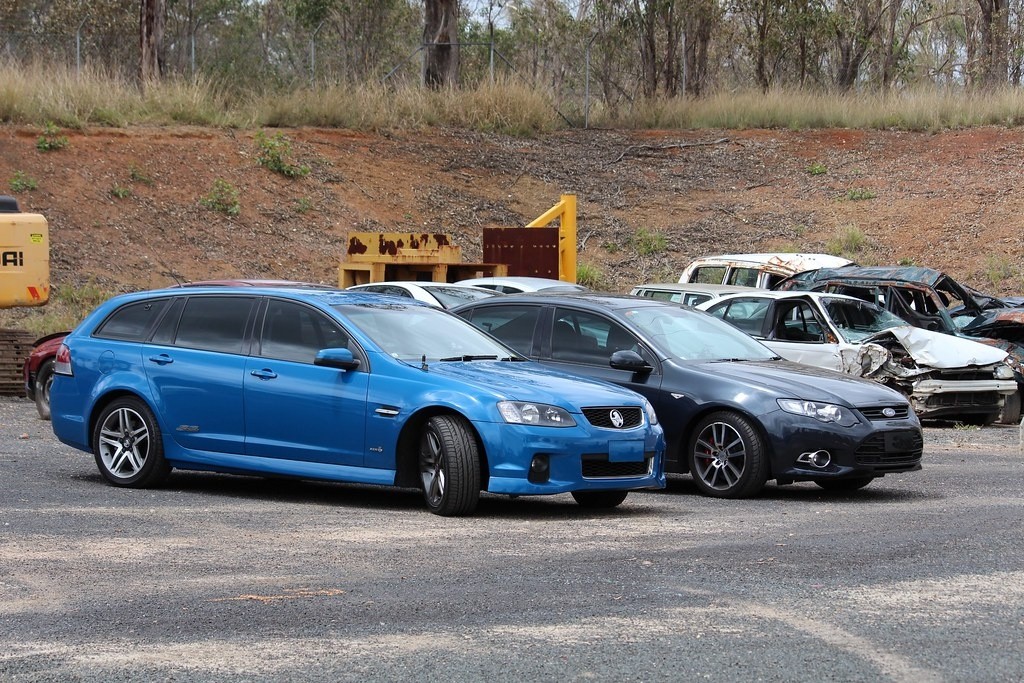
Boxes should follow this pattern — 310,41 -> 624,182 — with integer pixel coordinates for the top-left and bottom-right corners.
627,251 -> 1023,426
48,286 -> 667,518
23,279 -> 335,420
445,293 -> 924,500
452,276 -> 590,294
344,280 -> 504,310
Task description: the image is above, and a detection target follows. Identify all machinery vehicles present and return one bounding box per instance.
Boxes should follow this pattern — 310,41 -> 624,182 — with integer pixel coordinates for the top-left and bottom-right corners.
0,195 -> 50,399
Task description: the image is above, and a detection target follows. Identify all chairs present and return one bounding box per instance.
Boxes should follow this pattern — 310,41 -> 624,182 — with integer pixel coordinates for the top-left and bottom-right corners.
551,321 -> 579,351
606,327 -> 634,356
579,333 -> 597,353
270,317 -> 317,363
